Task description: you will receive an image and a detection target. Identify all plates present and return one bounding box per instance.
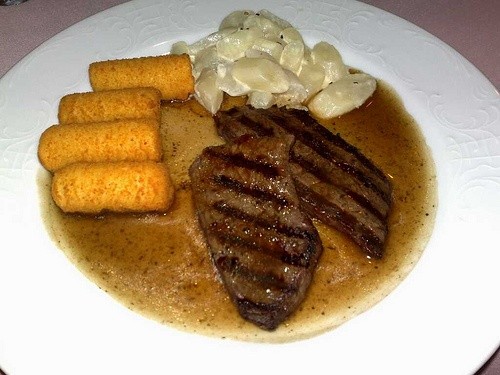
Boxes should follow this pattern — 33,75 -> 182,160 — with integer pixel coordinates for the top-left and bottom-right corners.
0,0 -> 500,375
